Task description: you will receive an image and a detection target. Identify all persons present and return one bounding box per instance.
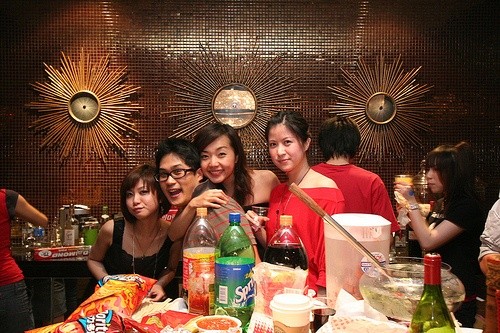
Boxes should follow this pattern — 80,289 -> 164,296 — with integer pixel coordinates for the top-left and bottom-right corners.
51,279 -> 66,325
479,195 -> 500,273
309,115 -> 400,249
0,189 -> 48,333
243,110 -> 344,288
156,139 -> 265,264
167,123 -> 281,259
393,144 -> 483,328
82,163 -> 187,301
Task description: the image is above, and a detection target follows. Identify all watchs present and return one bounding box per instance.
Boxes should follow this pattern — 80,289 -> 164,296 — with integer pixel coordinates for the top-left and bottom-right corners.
408,203 -> 418,210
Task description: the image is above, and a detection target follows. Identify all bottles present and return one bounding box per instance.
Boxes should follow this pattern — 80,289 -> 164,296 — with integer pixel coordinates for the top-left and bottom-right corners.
410,253 -> 456,333
214,213 -> 256,333
10,198 -> 113,248
425,200 -> 438,226
182,208 -> 220,317
406,223 -> 422,262
263,214 -> 308,297
357,252 -> 466,322
412,159 -> 434,215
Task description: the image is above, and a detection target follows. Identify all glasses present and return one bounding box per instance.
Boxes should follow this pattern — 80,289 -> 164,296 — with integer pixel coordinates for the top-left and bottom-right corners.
153,168 -> 196,182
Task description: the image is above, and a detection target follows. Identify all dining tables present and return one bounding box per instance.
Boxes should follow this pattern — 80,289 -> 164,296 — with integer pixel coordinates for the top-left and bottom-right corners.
24,276 -> 485,333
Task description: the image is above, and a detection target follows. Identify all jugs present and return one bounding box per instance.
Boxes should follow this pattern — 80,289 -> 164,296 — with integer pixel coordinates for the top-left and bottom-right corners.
323,212 -> 391,306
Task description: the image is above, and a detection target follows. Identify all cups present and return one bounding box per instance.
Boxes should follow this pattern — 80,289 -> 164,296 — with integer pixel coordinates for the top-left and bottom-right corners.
269,294 -> 313,333
252,206 -> 269,228
309,296 -> 336,333
485,254 -> 500,333
395,174 -> 413,206
193,315 -> 242,333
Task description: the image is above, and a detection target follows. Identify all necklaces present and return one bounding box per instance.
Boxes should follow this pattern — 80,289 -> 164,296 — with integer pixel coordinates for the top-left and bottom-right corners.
133,224 -> 158,260
133,226 -> 157,280
274,167 -> 310,233
